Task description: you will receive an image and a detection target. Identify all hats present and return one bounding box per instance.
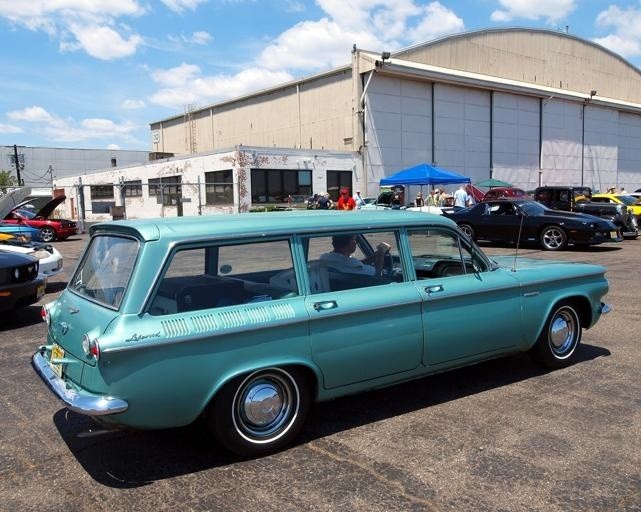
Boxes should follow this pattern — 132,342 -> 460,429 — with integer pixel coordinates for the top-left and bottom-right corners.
340,188 -> 349,194
435,189 -> 439,193
356,189 -> 361,193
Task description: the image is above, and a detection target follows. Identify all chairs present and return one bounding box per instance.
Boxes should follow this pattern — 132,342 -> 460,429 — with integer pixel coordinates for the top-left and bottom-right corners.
201,267 -> 394,300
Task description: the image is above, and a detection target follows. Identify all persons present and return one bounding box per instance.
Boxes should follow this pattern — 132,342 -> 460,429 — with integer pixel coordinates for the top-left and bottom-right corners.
390,187 -> 404,209
353,190 -> 367,209
425,190 -> 436,206
438,188 -> 448,205
307,193 -> 334,210
454,186 -> 468,209
434,190 -> 441,205
416,192 -> 425,207
338,189 -> 357,210
320,235 -> 391,282
